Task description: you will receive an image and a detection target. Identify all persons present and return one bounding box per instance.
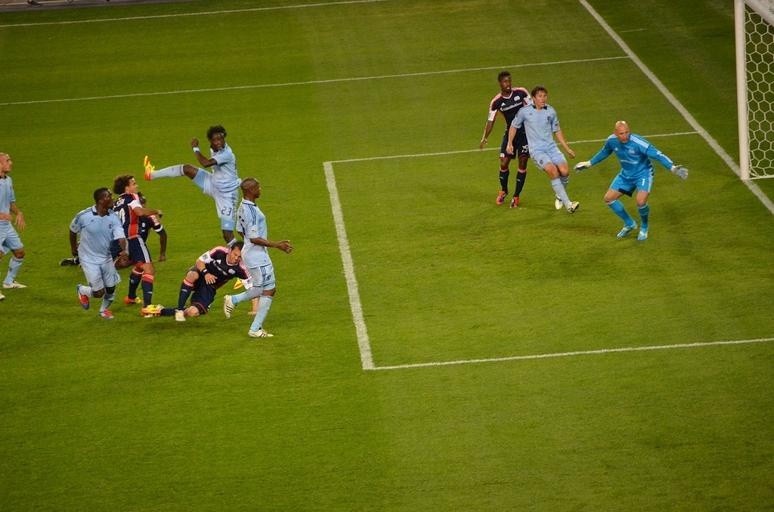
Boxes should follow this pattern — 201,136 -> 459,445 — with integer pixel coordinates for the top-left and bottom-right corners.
479,71 -> 534,208
506,86 -> 579,214
573,120 -> 689,242
141,240 -> 259,322
143,125 -> 244,290
60,174 -> 167,319
223,177 -> 295,338
0,152 -> 27,300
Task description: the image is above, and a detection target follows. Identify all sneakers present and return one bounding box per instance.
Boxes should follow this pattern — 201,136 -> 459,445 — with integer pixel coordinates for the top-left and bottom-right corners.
510,197 -> 520,208
2,280 -> 27,289
140,304 -> 164,317
247,326 -> 274,337
143,155 -> 156,181
99,309 -> 114,320
637,227 -> 649,241
495,191 -> 508,205
124,297 -> 143,304
567,201 -> 580,213
76,284 -> 89,309
60,258 -> 79,266
223,295 -> 235,319
555,196 -> 564,209
617,220 -> 638,240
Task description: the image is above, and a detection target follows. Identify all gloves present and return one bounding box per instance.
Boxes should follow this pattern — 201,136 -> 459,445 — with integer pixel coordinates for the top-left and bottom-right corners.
573,160 -> 592,172
670,164 -> 689,180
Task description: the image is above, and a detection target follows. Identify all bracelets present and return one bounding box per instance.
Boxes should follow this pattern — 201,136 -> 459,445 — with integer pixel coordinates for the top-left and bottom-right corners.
200,268 -> 209,278
192,147 -> 200,153
